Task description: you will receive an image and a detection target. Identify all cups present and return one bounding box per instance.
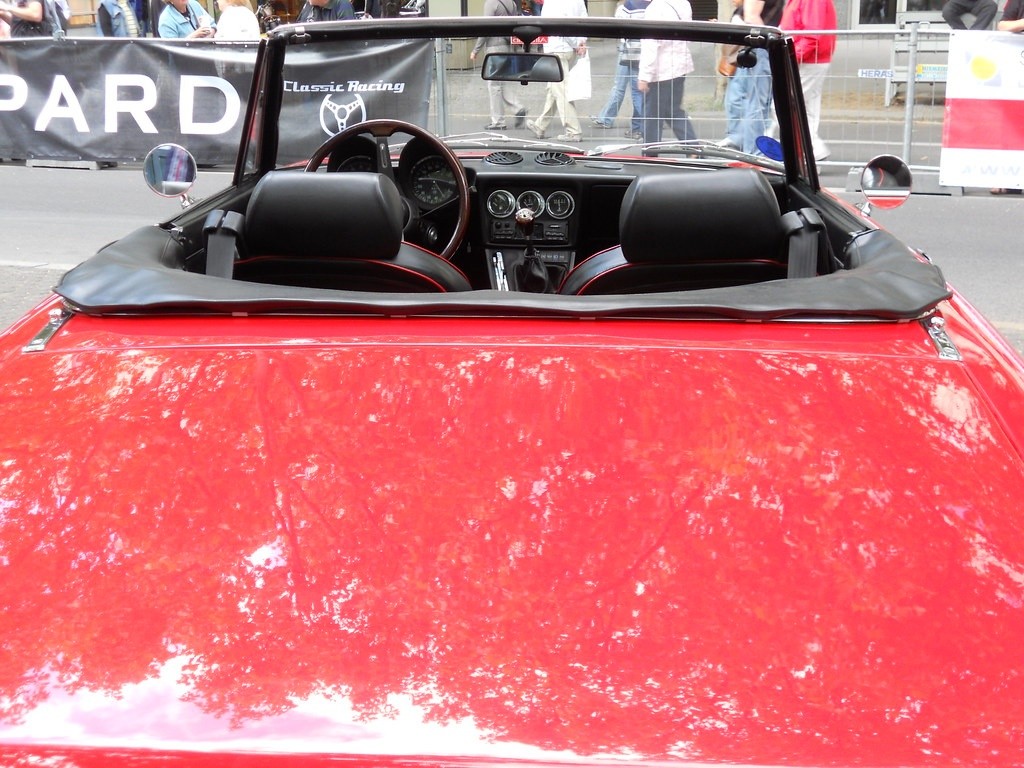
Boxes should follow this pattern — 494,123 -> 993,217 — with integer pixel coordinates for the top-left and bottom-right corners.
198,14 -> 212,31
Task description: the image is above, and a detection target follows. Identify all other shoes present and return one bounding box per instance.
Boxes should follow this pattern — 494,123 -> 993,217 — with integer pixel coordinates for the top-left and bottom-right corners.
485,123 -> 507,130
515,109 -> 528,128
687,146 -> 704,159
813,147 -> 831,160
593,119 -> 611,128
624,129 -> 643,139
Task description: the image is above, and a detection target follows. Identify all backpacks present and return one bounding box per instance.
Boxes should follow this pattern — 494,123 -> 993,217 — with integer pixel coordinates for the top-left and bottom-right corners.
44,0 -> 70,39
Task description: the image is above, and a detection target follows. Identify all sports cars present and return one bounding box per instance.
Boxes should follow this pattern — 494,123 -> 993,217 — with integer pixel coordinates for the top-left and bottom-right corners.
0,15 -> 1024,768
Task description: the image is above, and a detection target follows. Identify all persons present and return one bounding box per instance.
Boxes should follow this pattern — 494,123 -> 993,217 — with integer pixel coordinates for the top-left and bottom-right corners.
713,0 -> 784,158
638,0 -> 705,160
158,0 -> 217,38
95,0 -> 147,38
989,0 -> 1024,195
521,0 -> 592,142
764,0 -> 837,175
470,0 -> 527,129
0,0 -> 71,38
361,12 -> 373,19
865,0 -> 882,24
300,0 -> 354,22
591,0 -> 650,141
942,0 -> 998,30
213,0 -> 261,44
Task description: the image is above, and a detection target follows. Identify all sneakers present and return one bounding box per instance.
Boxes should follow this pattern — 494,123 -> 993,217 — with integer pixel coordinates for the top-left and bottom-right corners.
558,132 -> 582,142
526,119 -> 545,138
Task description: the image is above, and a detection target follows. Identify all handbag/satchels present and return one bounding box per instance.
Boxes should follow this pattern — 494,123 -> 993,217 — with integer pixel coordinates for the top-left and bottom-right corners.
719,43 -> 739,78
619,38 -> 641,66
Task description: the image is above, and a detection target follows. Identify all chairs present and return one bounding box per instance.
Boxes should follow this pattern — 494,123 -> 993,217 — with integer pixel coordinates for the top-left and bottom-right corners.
233,171 -> 471,293
557,168 -> 821,296
